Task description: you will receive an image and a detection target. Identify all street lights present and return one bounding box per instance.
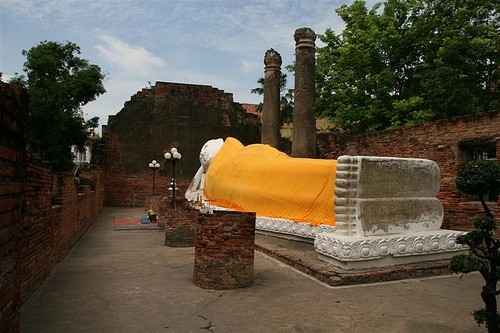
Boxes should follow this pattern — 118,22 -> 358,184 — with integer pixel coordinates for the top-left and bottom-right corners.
164,148 -> 181,207
149,160 -> 161,196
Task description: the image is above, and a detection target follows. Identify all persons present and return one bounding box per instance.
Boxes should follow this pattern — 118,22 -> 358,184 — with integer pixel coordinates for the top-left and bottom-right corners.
183,136 -> 444,237
147,208 -> 156,223
